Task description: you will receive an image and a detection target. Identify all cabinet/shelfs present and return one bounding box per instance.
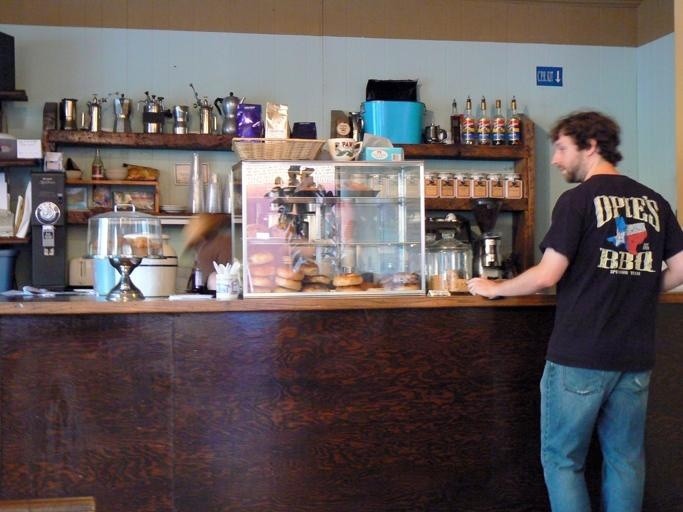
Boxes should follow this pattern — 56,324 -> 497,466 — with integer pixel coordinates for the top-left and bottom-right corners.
40,101 -> 536,292
229,158 -> 425,298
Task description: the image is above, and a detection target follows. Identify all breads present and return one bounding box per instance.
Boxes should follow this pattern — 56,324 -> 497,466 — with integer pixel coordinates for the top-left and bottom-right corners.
430,269 -> 468,291
92,236 -> 163,255
248,250 -> 420,292
310,182 -> 369,190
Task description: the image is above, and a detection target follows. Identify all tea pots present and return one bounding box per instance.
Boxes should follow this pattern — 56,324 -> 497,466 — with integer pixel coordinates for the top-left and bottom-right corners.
214,91 -> 246,135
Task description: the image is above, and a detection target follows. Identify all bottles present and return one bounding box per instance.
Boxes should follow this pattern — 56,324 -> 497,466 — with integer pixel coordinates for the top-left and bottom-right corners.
450,95 -> 523,145
191,152 -> 232,214
333,170 -> 523,199
194,267 -> 205,289
425,229 -> 473,293
91,146 -> 104,180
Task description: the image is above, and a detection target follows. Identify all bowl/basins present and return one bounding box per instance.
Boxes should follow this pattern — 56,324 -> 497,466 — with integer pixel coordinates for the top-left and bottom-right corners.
104,167 -> 129,180
65,170 -> 82,179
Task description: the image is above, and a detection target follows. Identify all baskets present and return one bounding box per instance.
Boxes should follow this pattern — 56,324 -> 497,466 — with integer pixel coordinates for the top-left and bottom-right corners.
231,137 -> 325,160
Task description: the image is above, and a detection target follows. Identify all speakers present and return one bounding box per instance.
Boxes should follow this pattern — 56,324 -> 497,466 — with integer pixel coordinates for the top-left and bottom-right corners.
1,31 -> 15,90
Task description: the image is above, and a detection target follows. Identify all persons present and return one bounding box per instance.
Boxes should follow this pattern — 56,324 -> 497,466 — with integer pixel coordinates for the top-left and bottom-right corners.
465,110 -> 683,511
180,178 -> 355,278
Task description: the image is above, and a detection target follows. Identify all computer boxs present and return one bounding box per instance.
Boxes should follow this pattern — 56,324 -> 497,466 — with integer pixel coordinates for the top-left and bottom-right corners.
31,172 -> 67,290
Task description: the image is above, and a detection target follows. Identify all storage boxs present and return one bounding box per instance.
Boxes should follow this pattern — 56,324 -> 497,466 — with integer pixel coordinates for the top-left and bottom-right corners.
360,100 -> 425,145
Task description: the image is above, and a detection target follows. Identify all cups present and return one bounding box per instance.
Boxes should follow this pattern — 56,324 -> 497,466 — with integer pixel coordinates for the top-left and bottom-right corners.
421,122 -> 447,144
215,274 -> 240,301
326,137 -> 364,161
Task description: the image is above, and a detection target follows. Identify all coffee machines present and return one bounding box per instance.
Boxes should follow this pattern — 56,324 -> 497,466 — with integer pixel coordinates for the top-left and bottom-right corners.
469,198 -> 508,279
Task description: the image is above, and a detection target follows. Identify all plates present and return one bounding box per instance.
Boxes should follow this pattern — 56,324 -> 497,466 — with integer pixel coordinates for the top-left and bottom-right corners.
160,204 -> 188,214
